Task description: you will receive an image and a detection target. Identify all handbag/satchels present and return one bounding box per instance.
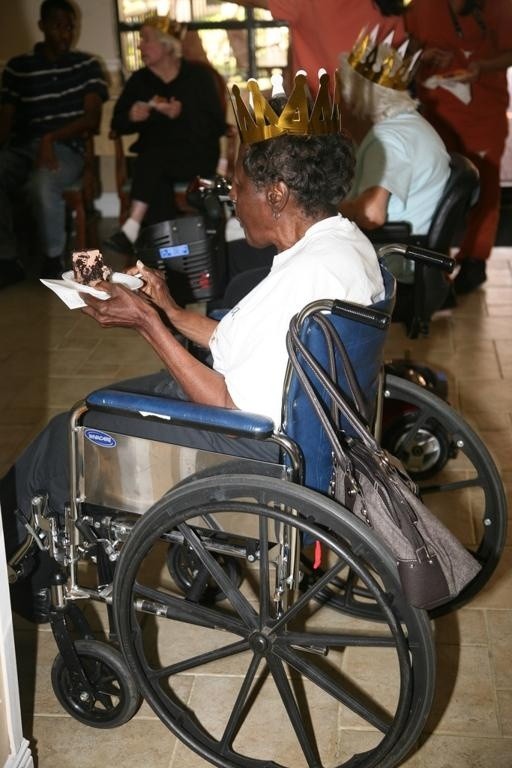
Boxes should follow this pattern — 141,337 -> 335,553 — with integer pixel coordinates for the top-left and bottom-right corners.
285,314 -> 483,610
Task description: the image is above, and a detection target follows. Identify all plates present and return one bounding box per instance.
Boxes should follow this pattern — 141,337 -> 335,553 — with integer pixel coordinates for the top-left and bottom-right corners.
61,266 -> 146,294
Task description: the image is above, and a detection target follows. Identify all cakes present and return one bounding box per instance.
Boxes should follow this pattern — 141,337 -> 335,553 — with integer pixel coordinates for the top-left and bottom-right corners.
71,250 -> 112,288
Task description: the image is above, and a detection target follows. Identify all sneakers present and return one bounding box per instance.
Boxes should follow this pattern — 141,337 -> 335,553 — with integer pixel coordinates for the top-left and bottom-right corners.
9,580 -> 55,624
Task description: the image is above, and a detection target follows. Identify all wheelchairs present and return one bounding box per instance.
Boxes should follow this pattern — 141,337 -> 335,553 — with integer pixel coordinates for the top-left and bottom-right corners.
185,153 -> 481,481
8,241 -> 508,768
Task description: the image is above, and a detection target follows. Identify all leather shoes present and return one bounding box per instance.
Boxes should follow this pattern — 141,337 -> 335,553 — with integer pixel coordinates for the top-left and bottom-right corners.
104,231 -> 138,256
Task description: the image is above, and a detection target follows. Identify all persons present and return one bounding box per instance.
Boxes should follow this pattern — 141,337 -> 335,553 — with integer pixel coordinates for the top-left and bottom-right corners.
102,18 -> 231,255
3,0 -> 109,285
324,43 -> 451,284
6,91 -> 387,622
